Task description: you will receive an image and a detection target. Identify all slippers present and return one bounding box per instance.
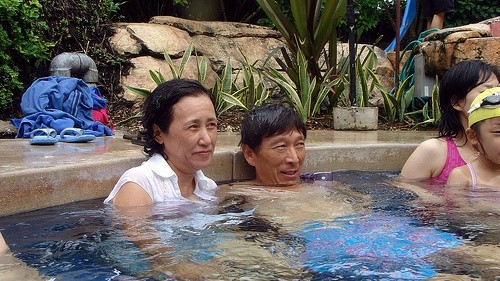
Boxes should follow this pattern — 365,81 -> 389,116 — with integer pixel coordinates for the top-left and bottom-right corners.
30,129 -> 58,145
59,127 -> 94,143
123,127 -> 139,138
131,130 -> 148,146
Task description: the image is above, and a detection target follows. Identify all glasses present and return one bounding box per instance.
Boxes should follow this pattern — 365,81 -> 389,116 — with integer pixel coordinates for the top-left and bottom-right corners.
466,95 -> 500,116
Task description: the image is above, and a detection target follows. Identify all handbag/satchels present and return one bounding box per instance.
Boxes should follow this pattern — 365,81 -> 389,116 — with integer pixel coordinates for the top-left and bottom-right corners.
91,96 -> 115,130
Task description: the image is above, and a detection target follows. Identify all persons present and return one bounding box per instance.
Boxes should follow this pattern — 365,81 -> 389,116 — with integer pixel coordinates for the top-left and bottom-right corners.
445,87 -> 500,215
103,78 -> 301,281
218,101 -> 500,281
420,0 -> 455,35
0,232 -> 55,281
394,59 -> 500,226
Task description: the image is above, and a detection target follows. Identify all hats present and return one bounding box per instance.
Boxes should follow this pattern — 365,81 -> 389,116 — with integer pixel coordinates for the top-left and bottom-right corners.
468,88 -> 500,127
